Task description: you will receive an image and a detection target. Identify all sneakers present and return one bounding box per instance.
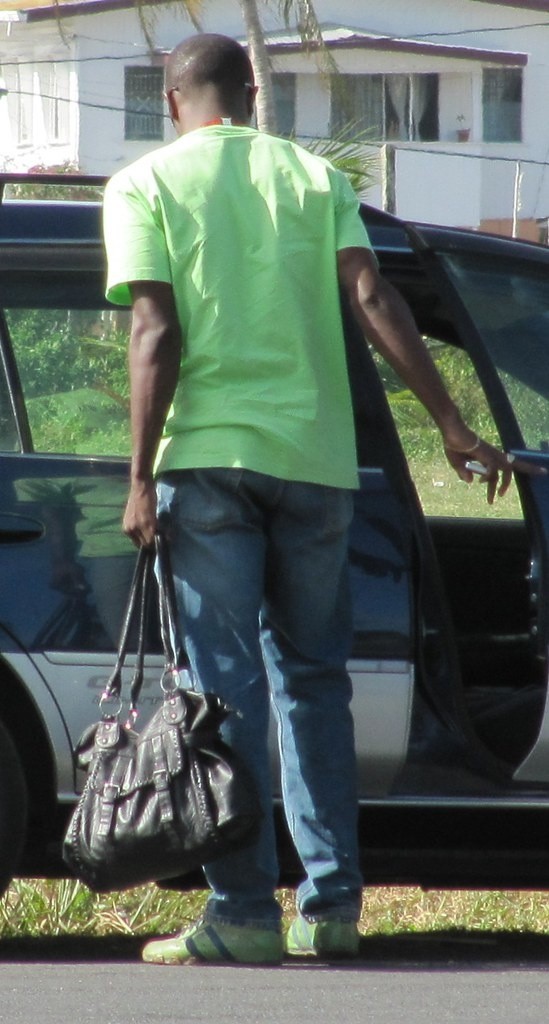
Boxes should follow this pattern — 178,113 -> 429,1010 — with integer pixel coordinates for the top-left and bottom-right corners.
141,919 -> 282,966
283,920 -> 360,956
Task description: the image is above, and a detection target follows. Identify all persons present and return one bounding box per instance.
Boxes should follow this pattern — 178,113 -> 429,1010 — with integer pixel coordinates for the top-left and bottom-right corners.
102,32 -> 513,968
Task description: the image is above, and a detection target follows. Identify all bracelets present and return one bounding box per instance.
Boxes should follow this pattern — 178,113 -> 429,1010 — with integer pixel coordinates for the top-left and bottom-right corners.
444,433 -> 479,453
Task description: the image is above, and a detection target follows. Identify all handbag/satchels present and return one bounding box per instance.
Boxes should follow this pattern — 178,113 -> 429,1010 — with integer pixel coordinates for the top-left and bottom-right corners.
61,533 -> 257,891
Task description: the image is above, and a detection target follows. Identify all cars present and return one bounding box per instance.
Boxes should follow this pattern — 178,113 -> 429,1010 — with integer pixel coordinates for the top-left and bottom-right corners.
0,169 -> 549,900
